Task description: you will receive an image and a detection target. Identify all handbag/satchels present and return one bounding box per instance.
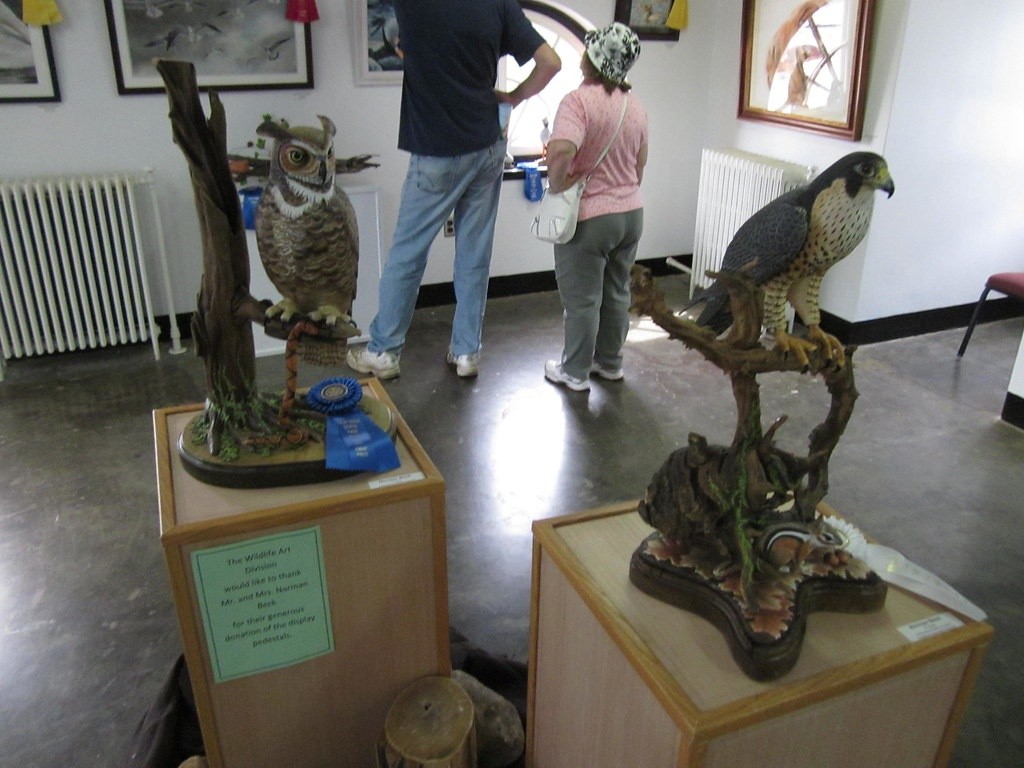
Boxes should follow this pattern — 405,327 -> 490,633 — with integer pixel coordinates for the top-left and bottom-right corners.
528,178 -> 585,243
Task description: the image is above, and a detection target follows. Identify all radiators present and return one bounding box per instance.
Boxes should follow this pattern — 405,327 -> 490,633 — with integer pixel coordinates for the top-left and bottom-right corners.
667,145 -> 817,339
2,167 -> 187,381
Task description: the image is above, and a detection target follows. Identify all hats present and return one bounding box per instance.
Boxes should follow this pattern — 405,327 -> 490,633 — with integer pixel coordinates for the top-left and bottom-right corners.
582,18 -> 640,84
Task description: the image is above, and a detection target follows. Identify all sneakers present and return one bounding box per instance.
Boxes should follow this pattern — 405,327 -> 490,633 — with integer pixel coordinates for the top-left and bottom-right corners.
346,347 -> 400,380
590,360 -> 625,382
543,360 -> 591,391
446,349 -> 481,377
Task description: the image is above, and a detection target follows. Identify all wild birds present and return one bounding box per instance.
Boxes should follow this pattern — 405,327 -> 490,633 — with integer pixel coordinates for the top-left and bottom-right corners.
666,152 -> 895,376
215,0 -> 256,20
166,22 -> 222,51
252,36 -> 292,61
168,0 -> 207,14
125,0 -> 171,18
142,36 -> 178,50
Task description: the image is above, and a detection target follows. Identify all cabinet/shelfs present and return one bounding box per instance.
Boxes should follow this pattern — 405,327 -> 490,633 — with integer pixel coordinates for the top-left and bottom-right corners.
148,371 -> 449,766
523,492 -> 999,768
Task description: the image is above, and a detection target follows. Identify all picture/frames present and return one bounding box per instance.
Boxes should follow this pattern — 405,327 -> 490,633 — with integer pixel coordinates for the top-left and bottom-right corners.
613,0 -> 682,45
0,2 -> 66,104
101,1 -> 316,97
736,0 -> 877,143
352,1 -> 406,87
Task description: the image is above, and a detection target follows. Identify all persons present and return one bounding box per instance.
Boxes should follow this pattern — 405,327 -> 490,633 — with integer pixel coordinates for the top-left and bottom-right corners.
543,25 -> 649,392
345,1 -> 564,380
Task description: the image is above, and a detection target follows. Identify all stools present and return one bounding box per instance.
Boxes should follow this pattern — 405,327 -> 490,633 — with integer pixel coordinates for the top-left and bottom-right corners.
956,272 -> 1023,362
